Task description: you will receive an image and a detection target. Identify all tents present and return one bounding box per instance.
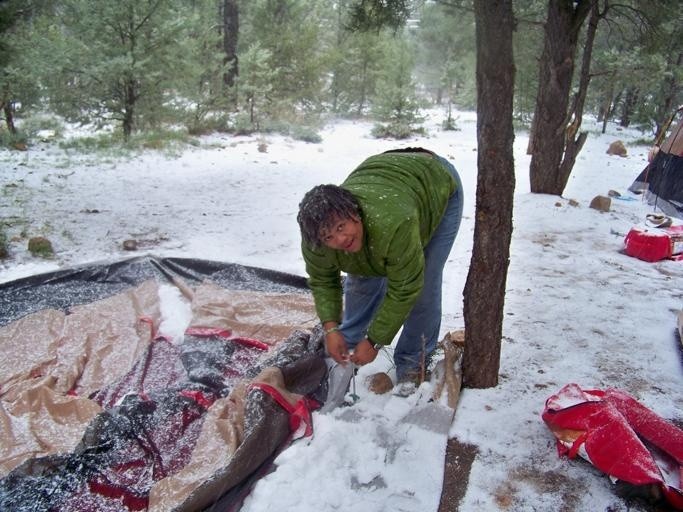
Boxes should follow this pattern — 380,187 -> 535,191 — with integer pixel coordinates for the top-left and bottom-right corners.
628,115 -> 683,219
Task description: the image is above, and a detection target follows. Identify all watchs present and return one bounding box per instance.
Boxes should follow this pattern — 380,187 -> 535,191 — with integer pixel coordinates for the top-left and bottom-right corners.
366,335 -> 383,351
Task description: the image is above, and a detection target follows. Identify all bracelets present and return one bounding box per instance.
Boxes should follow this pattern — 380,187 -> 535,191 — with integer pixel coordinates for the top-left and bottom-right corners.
324,327 -> 339,336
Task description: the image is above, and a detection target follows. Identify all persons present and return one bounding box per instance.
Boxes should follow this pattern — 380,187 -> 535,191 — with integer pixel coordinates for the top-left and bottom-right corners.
301,146 -> 464,397
